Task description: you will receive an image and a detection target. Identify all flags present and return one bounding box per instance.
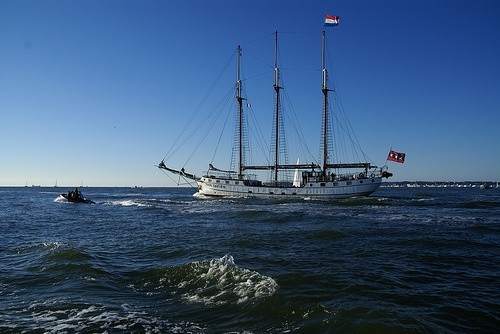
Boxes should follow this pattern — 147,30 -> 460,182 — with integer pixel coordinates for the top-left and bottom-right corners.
323,14 -> 340,27
387,150 -> 406,163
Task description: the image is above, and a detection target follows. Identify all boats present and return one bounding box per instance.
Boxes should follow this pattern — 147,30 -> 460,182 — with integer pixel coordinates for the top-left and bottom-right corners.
59,193 -> 96,205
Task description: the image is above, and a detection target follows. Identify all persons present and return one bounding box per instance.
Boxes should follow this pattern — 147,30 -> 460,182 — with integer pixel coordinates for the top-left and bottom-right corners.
68,188 -> 84,198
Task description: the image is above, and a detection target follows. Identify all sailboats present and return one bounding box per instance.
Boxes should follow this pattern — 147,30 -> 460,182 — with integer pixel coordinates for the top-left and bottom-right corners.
154,13 -> 406,200
